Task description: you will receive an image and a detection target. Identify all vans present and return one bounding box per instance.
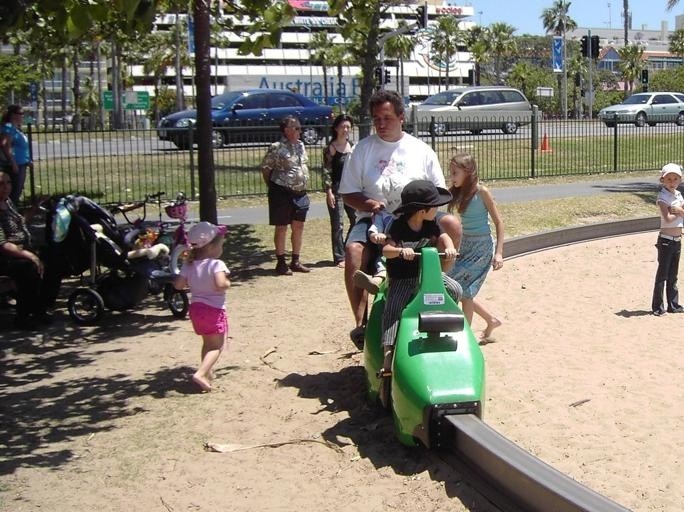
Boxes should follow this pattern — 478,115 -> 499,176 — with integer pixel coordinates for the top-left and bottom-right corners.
403,85 -> 534,137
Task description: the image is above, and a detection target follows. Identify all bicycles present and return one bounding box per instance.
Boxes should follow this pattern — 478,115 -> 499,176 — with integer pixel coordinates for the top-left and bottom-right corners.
166,189 -> 194,275
101,188 -> 169,296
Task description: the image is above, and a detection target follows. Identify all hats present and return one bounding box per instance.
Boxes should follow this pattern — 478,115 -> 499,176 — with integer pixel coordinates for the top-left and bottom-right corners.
662,163 -> 682,178
187,221 -> 228,249
393,179 -> 453,214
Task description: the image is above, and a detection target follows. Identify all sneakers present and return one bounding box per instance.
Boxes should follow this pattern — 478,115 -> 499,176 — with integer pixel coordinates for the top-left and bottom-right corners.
333,260 -> 345,268
276,261 -> 310,275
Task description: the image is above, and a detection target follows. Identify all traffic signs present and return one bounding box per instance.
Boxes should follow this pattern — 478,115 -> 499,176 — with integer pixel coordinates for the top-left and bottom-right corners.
102,91 -> 150,110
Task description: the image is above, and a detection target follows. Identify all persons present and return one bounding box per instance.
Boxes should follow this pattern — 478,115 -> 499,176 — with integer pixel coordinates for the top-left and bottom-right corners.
446,153 -> 504,339
261,115 -> 311,275
336,90 -> 461,352
320,113 -> 357,268
380,181 -> 463,369
0,171 -> 65,321
650,163 -> 684,316
173,222 -> 231,391
0,105 -> 34,204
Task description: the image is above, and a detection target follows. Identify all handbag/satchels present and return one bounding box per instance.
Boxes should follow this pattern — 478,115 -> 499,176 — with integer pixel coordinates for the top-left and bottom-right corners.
292,193 -> 310,215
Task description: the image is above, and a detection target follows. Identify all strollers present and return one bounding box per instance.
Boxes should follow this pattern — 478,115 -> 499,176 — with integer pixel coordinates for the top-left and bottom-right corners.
29,195 -> 190,325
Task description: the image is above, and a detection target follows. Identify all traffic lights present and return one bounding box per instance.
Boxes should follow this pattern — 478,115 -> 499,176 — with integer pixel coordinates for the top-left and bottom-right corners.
579,36 -> 588,58
414,4 -> 428,30
642,70 -> 648,83
590,35 -> 600,59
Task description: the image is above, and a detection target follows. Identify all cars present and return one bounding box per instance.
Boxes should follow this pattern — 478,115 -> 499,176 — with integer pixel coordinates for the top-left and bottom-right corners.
596,91 -> 684,128
155,87 -> 336,153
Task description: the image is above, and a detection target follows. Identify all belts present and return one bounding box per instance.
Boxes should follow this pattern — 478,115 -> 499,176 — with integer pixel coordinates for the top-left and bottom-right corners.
658,232 -> 682,242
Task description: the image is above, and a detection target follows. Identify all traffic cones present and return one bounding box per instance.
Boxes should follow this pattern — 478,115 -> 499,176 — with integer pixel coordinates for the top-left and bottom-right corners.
539,133 -> 550,152
